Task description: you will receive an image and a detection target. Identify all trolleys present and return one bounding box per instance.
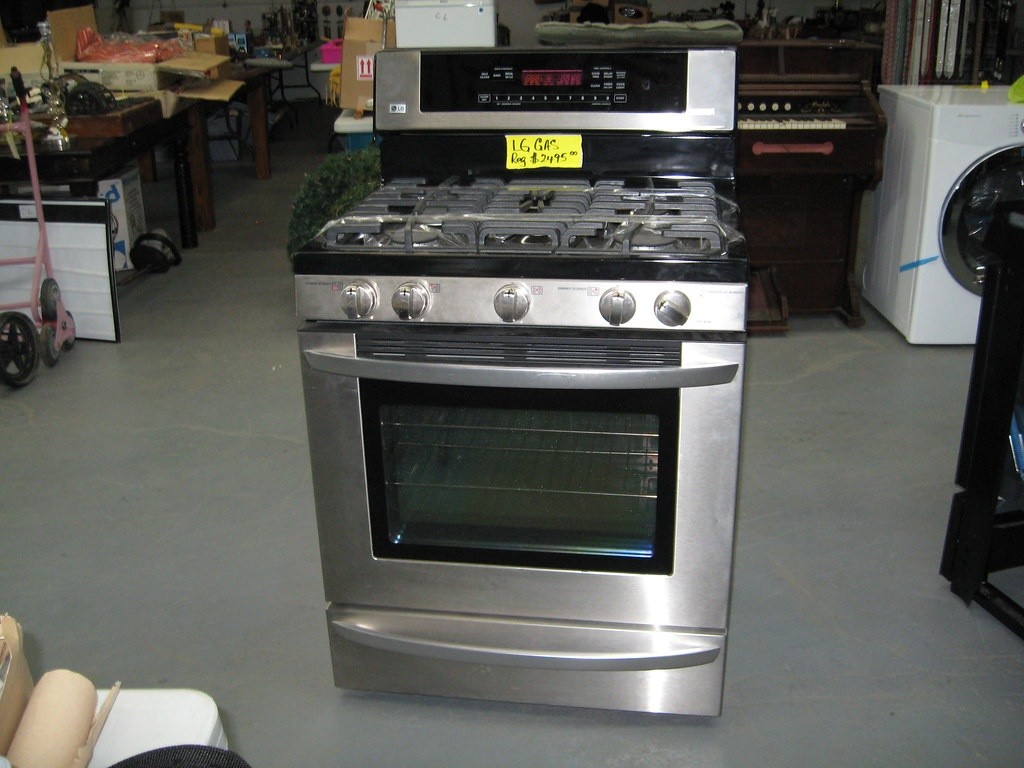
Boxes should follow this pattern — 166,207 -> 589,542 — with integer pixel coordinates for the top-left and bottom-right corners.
0,67 -> 79,390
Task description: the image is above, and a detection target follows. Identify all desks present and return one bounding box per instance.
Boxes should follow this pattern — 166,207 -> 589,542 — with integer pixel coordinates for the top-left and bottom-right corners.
0,65 -> 280,231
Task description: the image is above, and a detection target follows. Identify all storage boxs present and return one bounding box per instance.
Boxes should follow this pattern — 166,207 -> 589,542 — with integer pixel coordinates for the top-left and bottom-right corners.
319,37 -> 344,64
606,1 -> 650,25
245,39 -> 325,127
16,165 -> 145,271
191,34 -> 230,81
338,14 -> 396,111
46,3 -> 231,93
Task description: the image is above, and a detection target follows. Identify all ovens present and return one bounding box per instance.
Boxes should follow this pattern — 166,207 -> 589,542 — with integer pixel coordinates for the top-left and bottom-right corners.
291,327 -> 746,721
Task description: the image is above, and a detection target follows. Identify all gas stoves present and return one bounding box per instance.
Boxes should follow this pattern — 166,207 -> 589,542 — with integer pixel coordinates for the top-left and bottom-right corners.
286,46 -> 752,333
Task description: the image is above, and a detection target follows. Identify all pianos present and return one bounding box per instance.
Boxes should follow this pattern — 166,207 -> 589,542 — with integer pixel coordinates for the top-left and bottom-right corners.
734,73 -> 887,335
